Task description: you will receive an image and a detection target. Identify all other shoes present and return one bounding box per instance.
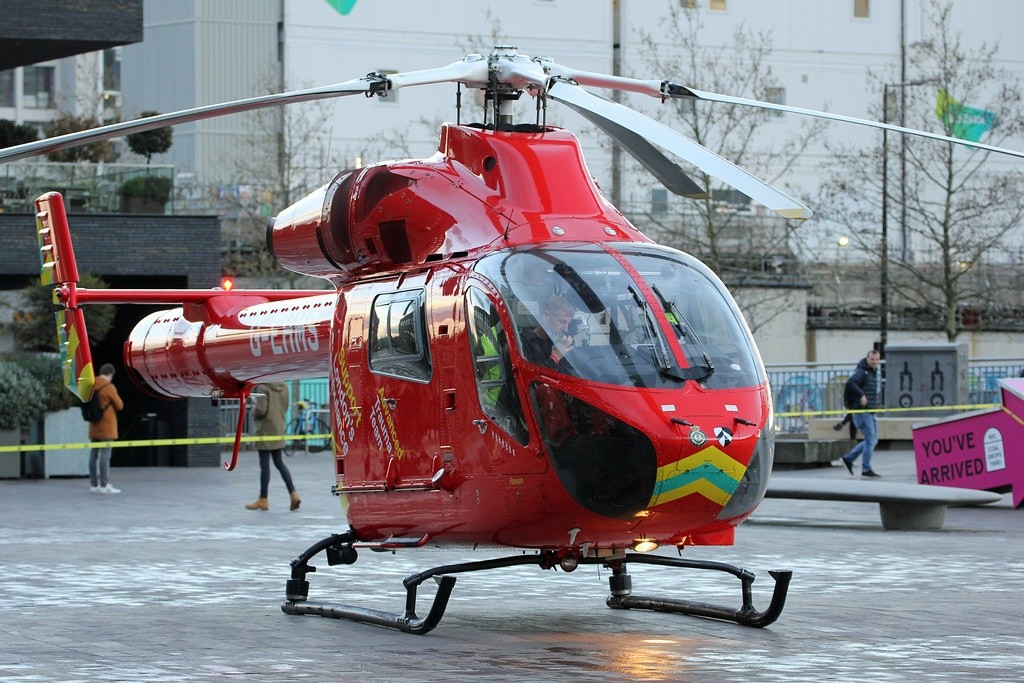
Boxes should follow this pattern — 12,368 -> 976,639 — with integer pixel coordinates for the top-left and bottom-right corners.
833,423 -> 842,431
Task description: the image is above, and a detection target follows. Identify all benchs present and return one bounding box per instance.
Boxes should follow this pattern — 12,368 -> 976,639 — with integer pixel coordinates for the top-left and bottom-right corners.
764,477 -> 1002,531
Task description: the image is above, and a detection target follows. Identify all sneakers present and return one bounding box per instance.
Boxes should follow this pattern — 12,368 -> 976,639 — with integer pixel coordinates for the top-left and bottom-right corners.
839,457 -> 854,476
90,484 -> 121,494
290,491 -> 301,511
245,498 -> 268,510
860,470 -> 882,479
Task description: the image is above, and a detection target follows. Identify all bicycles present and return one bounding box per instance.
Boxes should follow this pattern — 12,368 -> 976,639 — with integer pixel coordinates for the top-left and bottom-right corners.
283,397 -> 333,458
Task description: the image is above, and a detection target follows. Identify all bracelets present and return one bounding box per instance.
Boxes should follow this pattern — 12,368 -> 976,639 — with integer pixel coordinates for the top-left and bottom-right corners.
548,353 -> 560,365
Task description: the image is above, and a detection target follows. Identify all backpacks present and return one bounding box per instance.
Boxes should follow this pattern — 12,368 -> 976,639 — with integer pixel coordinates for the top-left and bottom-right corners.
844,370 -> 866,410
81,382 -> 111,423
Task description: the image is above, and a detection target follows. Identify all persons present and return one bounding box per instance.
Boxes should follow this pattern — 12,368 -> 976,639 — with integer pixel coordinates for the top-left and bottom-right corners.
501,295 -> 638,510
839,350 -> 883,478
88,364 -> 121,494
245,381 -> 302,512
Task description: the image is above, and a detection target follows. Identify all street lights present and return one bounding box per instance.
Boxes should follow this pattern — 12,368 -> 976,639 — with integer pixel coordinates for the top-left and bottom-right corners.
878,76 -> 929,406
835,236 -> 850,320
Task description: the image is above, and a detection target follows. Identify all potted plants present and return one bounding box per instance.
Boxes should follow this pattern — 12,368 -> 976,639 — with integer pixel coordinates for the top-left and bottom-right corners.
118,110 -> 173,214
41,117 -> 120,214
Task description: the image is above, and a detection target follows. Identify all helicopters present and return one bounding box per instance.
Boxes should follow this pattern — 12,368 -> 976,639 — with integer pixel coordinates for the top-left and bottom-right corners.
0,42 -> 1024,637
